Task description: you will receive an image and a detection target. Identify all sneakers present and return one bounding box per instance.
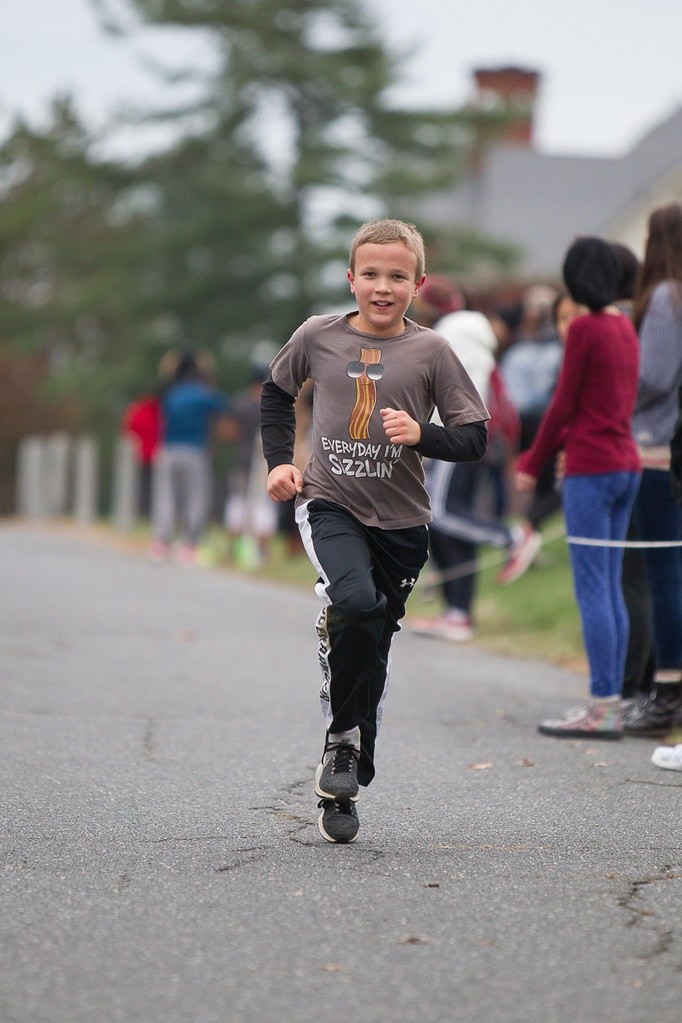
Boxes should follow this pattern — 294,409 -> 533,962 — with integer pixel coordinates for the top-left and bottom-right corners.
652,744 -> 681,772
317,798 -> 363,849
316,726 -> 366,805
410,606 -> 474,642
616,697 -> 678,738
540,694 -> 624,744
497,525 -> 543,582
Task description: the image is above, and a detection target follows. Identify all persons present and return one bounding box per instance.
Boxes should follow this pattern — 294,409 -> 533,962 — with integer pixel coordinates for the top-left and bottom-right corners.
127,335 -> 308,578
618,201 -> 681,739
467,283 -> 589,566
260,219 -> 493,843
406,273 -> 541,643
512,233 -> 657,741
